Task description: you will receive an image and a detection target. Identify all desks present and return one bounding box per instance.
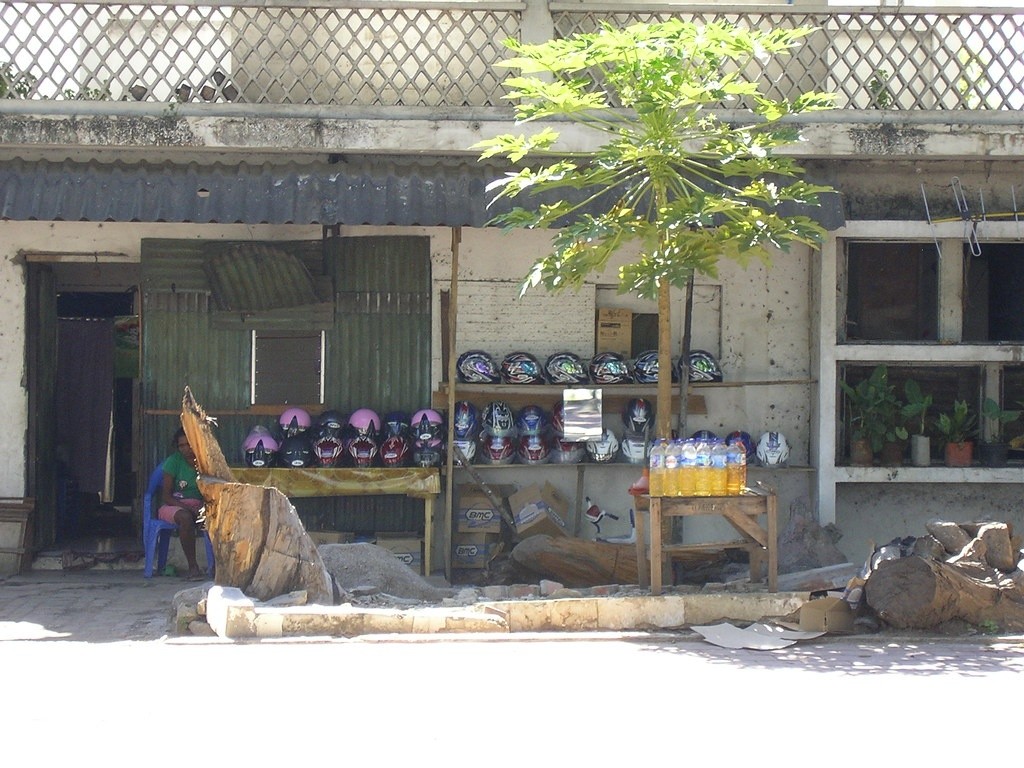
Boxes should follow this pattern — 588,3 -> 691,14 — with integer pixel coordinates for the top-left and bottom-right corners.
634,481 -> 778,593
224,465 -> 440,576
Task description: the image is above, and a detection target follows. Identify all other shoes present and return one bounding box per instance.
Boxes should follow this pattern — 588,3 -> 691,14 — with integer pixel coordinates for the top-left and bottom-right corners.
186,571 -> 203,581
204,567 -> 215,580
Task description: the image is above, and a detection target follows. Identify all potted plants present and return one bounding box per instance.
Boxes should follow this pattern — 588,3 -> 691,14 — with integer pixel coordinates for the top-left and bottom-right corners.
901,380 -> 933,466
880,416 -> 907,466
839,367 -> 894,466
979,397 -> 1020,467
935,400 -> 977,466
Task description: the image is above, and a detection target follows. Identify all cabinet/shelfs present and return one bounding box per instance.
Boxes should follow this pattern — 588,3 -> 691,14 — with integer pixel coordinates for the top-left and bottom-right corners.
452,377 -> 820,472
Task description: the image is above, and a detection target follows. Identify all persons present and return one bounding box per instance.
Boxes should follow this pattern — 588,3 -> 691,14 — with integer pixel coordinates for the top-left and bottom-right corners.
157,424 -> 207,582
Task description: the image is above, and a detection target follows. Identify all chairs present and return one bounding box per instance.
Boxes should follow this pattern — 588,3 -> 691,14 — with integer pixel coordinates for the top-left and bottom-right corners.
142,455 -> 215,579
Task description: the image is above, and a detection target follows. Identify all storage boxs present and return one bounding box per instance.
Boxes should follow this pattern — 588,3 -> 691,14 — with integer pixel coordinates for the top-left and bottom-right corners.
596,305 -> 633,360
457,483 -> 511,533
507,481 -> 571,539
452,533 -> 511,570
307,530 -> 358,550
371,532 -> 424,577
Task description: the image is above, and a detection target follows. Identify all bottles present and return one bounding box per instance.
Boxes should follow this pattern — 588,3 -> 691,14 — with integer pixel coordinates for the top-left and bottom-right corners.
650,436 -> 747,495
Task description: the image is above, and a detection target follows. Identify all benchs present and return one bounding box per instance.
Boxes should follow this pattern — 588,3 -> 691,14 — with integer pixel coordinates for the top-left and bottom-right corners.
0,496 -> 35,574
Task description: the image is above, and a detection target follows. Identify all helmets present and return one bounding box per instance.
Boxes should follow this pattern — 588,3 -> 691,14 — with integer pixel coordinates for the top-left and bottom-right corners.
678,350 -> 722,382
635,350 -> 678,383
545,351 -> 591,384
500,353 -> 545,385
238,396 -> 792,468
457,350 -> 501,384
590,352 -> 634,384
